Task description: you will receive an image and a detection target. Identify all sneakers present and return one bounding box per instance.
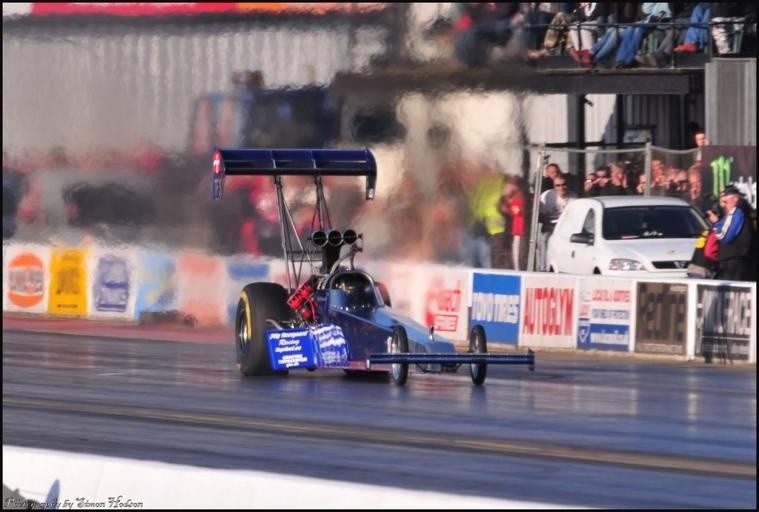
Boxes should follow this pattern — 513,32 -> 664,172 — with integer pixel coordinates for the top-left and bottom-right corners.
616,44 -> 697,68
569,48 -> 593,68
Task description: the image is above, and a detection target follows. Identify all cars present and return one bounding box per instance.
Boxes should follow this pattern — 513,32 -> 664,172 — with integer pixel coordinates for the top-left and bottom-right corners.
545,196 -> 712,273
209,146 -> 534,383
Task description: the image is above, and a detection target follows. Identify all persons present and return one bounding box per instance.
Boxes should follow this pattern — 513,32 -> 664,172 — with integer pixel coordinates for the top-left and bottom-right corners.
422,1 -> 758,74
379,131 -> 758,282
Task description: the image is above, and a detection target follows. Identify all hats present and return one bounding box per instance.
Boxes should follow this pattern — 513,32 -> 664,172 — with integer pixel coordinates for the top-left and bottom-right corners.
720,187 -> 745,196
